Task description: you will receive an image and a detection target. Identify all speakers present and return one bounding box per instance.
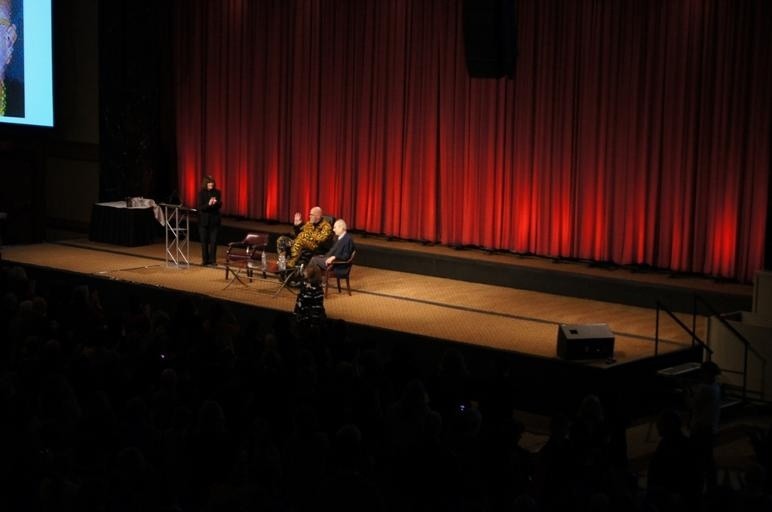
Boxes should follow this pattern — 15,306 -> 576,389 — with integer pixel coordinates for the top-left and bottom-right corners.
462,0 -> 518,79
558,324 -> 614,359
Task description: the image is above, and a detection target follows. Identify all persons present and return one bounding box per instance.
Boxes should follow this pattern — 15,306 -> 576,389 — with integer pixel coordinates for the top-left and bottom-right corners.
195,175 -> 224,267
0,261 -> 772,512
275,206 -> 333,280
294,216 -> 356,290
1,0 -> 24,117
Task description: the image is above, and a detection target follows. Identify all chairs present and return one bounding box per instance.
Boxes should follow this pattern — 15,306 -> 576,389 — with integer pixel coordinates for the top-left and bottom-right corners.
315,215 -> 336,255
322,248 -> 356,299
226,232 -> 269,282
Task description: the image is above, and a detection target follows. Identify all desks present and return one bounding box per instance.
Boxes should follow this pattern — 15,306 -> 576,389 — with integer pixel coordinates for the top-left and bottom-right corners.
221,259 -> 303,298
